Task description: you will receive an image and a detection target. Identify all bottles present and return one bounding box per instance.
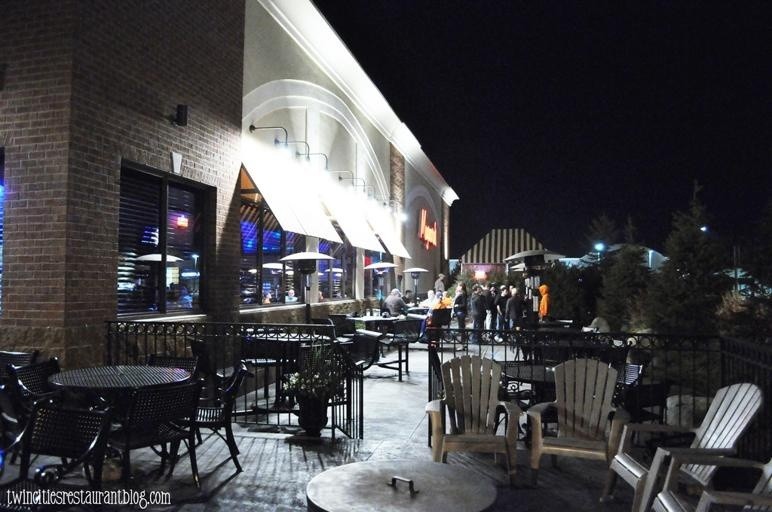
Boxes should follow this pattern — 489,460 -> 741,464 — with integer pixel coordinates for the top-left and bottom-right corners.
416,297 -> 420,304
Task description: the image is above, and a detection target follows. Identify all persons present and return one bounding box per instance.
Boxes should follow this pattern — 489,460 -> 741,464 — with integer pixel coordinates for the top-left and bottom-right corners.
166,273 -> 553,346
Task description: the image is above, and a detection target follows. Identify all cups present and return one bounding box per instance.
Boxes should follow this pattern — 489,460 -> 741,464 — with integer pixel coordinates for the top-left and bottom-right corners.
366,308 -> 381,317
